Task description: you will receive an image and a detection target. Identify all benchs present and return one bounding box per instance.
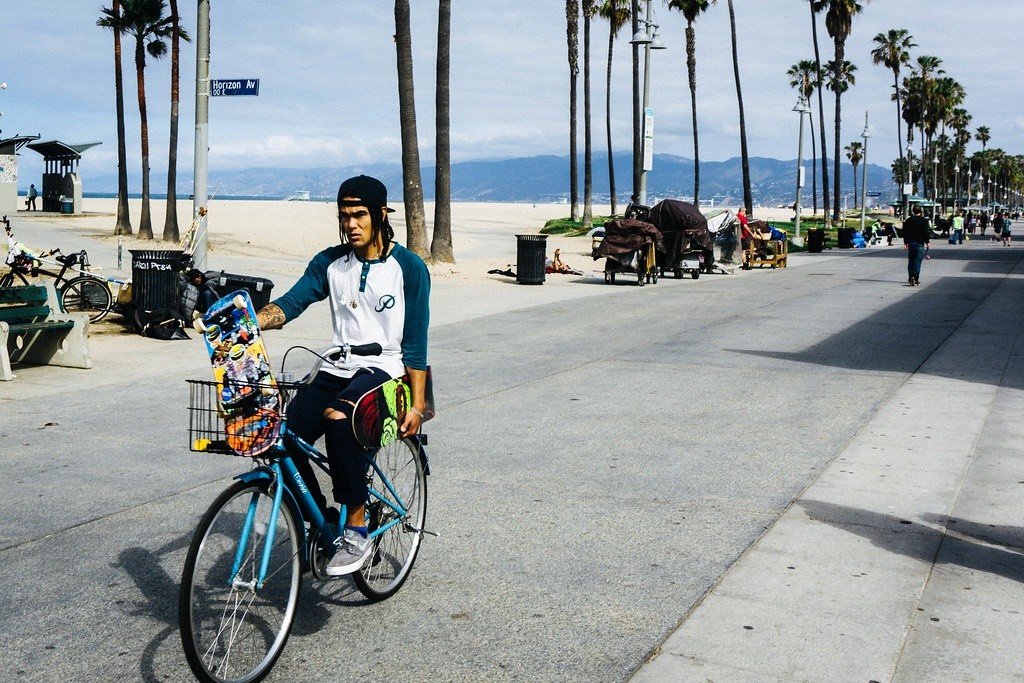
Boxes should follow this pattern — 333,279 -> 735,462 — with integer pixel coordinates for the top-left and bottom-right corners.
0,279 -> 94,380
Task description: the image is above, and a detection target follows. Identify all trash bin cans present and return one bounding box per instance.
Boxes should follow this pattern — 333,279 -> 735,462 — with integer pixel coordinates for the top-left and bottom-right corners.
836,227 -> 853,249
127,248 -> 185,318
807,228 -> 824,253
515,234 -> 550,285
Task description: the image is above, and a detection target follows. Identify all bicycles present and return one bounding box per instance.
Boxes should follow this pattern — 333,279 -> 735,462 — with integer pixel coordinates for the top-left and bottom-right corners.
176,342 -> 440,683
0,219 -> 113,324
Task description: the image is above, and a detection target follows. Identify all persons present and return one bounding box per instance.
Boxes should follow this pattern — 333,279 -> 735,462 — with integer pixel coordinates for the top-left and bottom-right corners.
26,184 -> 37,211
184,269 -> 221,320
924,209 -> 1020,246
902,205 -> 930,286
545,248 -> 571,271
871,219 -> 894,246
255,174 -> 432,577
735,207 -> 756,270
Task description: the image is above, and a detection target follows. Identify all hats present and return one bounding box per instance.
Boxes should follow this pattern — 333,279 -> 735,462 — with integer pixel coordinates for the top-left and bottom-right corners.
187,269 -> 201,282
337,174 -> 397,213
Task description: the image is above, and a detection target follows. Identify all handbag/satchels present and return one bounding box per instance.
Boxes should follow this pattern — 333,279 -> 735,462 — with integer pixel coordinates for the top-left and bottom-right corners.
923,247 -> 935,260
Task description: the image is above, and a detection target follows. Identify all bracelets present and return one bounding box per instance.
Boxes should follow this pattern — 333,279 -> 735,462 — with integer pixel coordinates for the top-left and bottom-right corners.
410,407 -> 425,419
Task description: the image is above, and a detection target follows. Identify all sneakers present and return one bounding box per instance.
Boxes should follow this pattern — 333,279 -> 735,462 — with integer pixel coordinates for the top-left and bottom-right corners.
325,525 -> 373,575
304,521 -> 310,540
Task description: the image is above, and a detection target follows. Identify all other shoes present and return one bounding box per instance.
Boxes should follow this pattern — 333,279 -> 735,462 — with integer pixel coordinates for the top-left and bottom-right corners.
910,277 -> 915,286
742,263 -> 752,270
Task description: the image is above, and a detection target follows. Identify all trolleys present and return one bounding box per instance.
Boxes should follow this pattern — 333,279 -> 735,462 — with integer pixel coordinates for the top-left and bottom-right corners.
603,204 -> 661,287
657,259 -> 701,279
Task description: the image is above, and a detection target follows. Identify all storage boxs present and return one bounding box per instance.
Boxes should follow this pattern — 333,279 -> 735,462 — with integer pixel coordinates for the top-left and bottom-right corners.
219,273 -> 274,313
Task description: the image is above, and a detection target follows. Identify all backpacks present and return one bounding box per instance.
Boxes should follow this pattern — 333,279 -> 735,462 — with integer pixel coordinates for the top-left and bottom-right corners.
968,227 -> 973,233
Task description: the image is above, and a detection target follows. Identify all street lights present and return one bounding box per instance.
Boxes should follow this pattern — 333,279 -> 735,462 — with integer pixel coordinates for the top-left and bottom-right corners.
630,18 -> 668,206
988,179 -> 1022,217
860,127 -> 871,227
905,141 -> 916,217
978,176 -> 983,215
954,165 -> 960,216
791,94 -> 811,235
967,171 -> 972,212
931,154 -> 940,227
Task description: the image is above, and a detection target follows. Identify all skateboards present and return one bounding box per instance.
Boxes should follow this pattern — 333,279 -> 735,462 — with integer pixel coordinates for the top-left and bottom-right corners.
192,288 -> 286,458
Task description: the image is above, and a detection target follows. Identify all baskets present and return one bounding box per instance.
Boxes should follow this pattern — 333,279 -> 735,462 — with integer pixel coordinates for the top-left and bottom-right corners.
185,379 -> 299,458
5,252 -> 43,275
